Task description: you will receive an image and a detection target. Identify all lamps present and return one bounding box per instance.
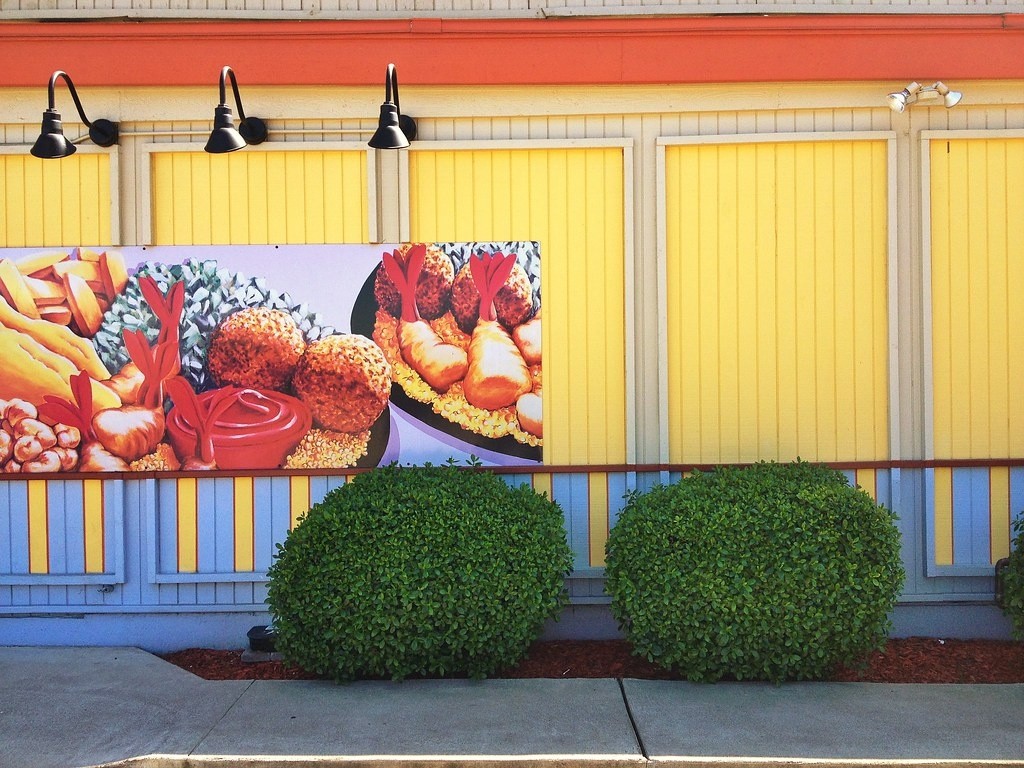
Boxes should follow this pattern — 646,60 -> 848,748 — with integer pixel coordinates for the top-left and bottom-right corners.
204,66 -> 267,154
30,70 -> 119,160
367,63 -> 417,150
931,81 -> 963,108
885,81 -> 919,114
